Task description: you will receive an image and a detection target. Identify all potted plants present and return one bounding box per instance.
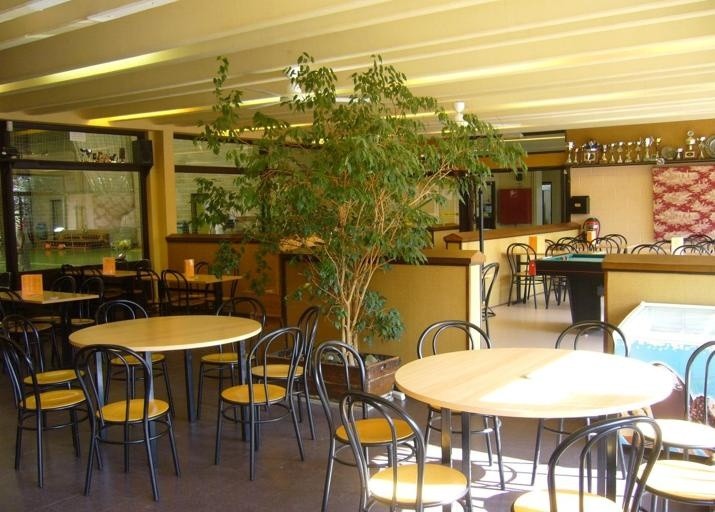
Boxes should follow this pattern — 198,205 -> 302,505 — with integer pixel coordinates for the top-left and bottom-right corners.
182,53 -> 529,406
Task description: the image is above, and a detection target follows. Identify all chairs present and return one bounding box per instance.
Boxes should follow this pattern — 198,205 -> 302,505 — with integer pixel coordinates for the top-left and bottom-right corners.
193,294 -> 272,426
528,319 -> 633,493
627,455 -> 715,512
505,413 -> 664,512
415,315 -> 508,493
92,300 -> 179,424
622,340 -> 715,508
210,325 -> 306,483
309,336 -> 423,511
1,337 -> 104,490
466,236 -> 715,340
1,234 -> 248,395
72,342 -> 184,503
336,390 -> 474,512
247,303 -> 323,441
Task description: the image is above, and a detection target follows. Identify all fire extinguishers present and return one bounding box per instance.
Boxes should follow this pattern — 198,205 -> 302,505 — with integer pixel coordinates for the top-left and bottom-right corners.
582,217 -> 600,245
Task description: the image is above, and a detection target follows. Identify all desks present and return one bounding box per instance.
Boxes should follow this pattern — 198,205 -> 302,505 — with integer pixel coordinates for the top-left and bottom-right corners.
392,345 -> 680,508
67,312 -> 265,455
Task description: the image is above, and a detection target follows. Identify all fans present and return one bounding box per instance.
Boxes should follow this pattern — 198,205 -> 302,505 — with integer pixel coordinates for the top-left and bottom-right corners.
240,67 -> 372,113
453,103 -> 472,130
